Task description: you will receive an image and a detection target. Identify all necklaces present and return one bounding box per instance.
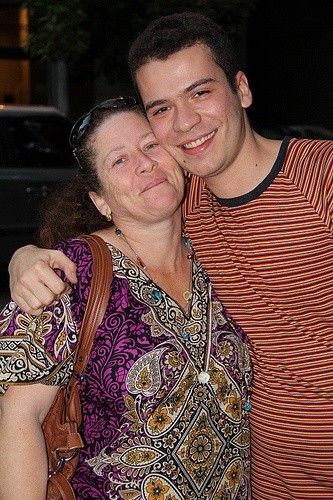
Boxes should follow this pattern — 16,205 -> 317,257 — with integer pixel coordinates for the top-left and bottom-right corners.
115,226 -> 195,312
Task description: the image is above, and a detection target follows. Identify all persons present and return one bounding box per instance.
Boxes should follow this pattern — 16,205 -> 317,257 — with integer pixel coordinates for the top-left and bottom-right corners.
8,11 -> 333,500
0,97 -> 254,500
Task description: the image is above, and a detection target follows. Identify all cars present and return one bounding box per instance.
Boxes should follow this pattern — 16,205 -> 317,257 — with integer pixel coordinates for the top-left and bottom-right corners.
0,103 -> 82,234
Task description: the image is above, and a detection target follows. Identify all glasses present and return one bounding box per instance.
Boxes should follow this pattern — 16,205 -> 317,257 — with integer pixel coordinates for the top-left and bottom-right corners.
68,97 -> 142,172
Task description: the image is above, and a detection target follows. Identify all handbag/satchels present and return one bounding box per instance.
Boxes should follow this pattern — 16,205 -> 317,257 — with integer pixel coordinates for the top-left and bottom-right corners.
39,234 -> 109,500
